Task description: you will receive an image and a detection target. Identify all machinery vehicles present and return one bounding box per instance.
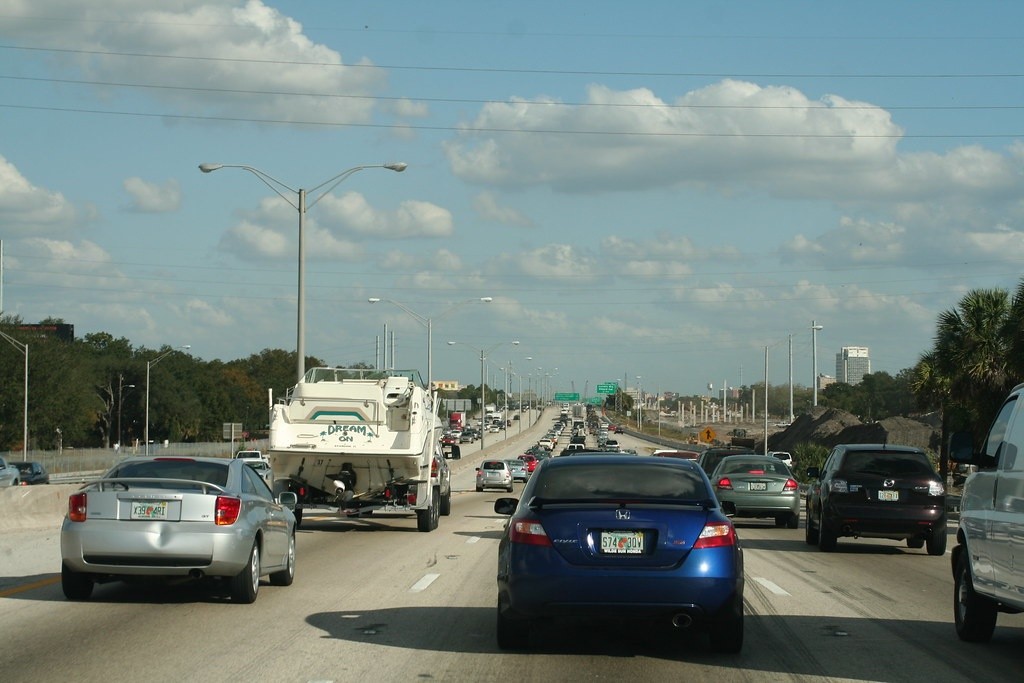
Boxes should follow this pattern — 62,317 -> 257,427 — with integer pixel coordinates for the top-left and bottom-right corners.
267,367 -> 453,534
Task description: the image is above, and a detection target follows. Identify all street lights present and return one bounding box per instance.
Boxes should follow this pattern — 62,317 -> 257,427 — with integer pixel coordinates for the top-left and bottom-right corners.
366,296 -> 493,396
447,341 -> 521,450
810,321 -> 824,407
143,344 -> 192,456
118,374 -> 136,447
196,162 -> 410,384
479,356 -> 558,439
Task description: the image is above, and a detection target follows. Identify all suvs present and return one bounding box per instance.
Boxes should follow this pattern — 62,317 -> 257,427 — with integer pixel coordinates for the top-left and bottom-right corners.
949,384 -> 1023,645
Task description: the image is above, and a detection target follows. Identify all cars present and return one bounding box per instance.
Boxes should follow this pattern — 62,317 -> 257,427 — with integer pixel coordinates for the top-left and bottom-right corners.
438,405 -> 530,447
693,446 -> 755,484
475,461 -> 515,494
492,456 -> 746,654
804,444 -> 949,556
0,455 -> 20,487
651,448 -> 701,466
768,452 -> 793,469
8,461 -> 51,487
518,401 -> 637,471
236,448 -> 273,481
504,459 -> 529,484
61,455 -> 301,604
704,454 -> 800,530
